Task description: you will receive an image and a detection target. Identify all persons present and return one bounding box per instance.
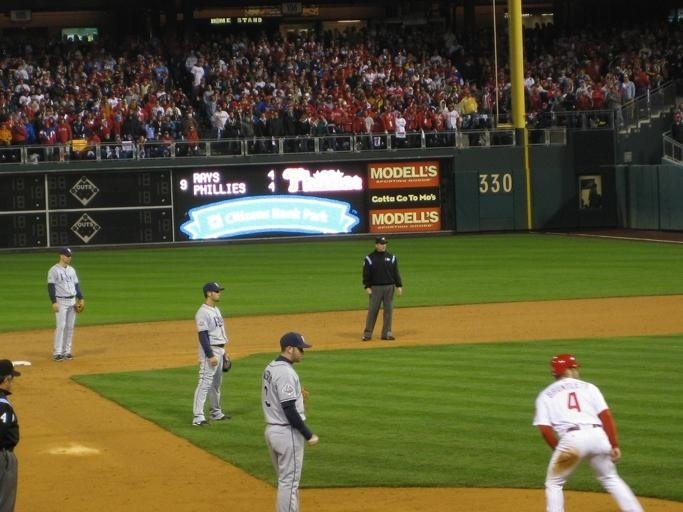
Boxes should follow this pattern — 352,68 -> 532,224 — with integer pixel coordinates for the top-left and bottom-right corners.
47,247 -> 85,361
192,281 -> 233,426
262,332 -> 319,512
531,354 -> 646,512
0,359 -> 22,512
0,20 -> 683,166
359,236 -> 404,342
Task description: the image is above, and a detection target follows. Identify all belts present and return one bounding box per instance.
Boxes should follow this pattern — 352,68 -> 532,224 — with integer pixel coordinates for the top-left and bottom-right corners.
56,296 -> 74,298
211,344 -> 224,347
567,424 -> 600,431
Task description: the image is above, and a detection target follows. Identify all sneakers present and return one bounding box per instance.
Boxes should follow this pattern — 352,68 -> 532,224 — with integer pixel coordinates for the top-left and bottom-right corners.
192,416 -> 231,426
382,337 -> 394,340
54,354 -> 72,361
363,337 -> 371,340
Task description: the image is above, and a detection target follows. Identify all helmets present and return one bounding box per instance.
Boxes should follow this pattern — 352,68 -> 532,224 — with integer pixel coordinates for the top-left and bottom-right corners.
551,354 -> 578,377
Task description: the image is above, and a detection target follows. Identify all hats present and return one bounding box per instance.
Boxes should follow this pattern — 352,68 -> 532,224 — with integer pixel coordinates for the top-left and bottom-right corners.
0,360 -> 20,376
376,236 -> 388,243
203,283 -> 224,291
280,333 -> 312,348
60,249 -> 72,256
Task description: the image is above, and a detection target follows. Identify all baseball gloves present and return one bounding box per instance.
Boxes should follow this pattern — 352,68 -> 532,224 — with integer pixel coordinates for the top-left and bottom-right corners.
223,355 -> 231,372
74,302 -> 86,312
